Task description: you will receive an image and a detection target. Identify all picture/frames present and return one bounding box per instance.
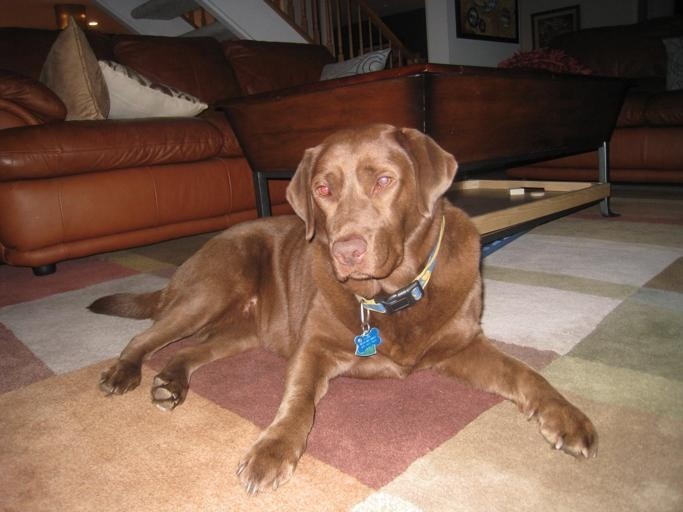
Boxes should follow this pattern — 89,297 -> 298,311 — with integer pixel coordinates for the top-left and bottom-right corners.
455,1 -> 520,43
531,5 -> 580,53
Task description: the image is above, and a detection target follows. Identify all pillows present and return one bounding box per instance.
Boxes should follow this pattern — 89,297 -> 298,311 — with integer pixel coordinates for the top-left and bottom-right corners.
36,14 -> 207,120
322,46 -> 391,81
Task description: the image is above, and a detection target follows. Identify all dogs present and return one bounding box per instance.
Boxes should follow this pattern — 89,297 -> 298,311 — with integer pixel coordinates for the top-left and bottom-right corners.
84,121 -> 599,497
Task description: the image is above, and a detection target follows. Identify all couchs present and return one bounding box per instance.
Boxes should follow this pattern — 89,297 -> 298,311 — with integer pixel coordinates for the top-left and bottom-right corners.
0,29 -> 337,275
504,17 -> 682,183
